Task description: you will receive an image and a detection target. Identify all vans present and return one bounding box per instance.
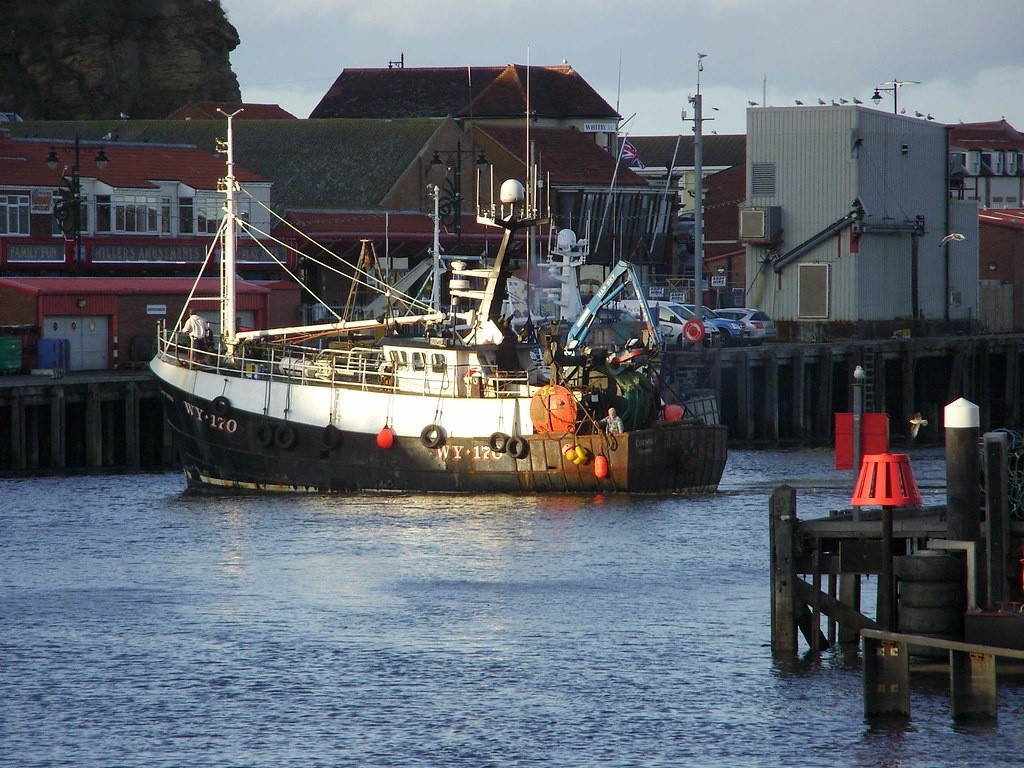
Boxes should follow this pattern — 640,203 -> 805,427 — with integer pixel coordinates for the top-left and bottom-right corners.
613,299 -> 722,352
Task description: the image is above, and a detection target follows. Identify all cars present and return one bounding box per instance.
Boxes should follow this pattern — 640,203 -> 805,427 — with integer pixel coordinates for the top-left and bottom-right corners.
0,111 -> 24,123
597,307 -> 640,343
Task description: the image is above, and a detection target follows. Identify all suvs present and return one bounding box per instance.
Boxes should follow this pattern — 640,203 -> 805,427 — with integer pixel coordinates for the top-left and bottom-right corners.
705,307 -> 777,346
678,303 -> 746,348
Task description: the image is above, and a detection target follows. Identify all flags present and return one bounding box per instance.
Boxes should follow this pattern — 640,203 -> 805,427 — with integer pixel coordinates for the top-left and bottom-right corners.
621,139 -> 645,170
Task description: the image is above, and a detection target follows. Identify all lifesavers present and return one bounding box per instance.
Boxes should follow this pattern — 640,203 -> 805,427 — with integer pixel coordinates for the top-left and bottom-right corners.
210,396 -> 231,416
896,555 -> 966,660
420,425 -> 442,448
507,437 -> 528,458
488,433 -> 507,452
322,425 -> 342,448
252,423 -> 274,445
274,424 -> 297,449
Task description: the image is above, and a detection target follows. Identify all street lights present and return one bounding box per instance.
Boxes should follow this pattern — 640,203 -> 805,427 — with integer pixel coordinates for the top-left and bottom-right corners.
428,134 -> 491,234
44,133 -> 112,277
388,51 -> 404,69
870,77 -> 898,113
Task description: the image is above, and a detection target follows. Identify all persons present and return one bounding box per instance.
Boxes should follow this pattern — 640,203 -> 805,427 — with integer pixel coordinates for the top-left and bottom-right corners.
599,407 -> 624,434
182,309 -> 205,357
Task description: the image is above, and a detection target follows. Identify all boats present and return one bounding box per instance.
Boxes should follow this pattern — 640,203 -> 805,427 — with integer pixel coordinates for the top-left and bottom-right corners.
147,107 -> 734,496
